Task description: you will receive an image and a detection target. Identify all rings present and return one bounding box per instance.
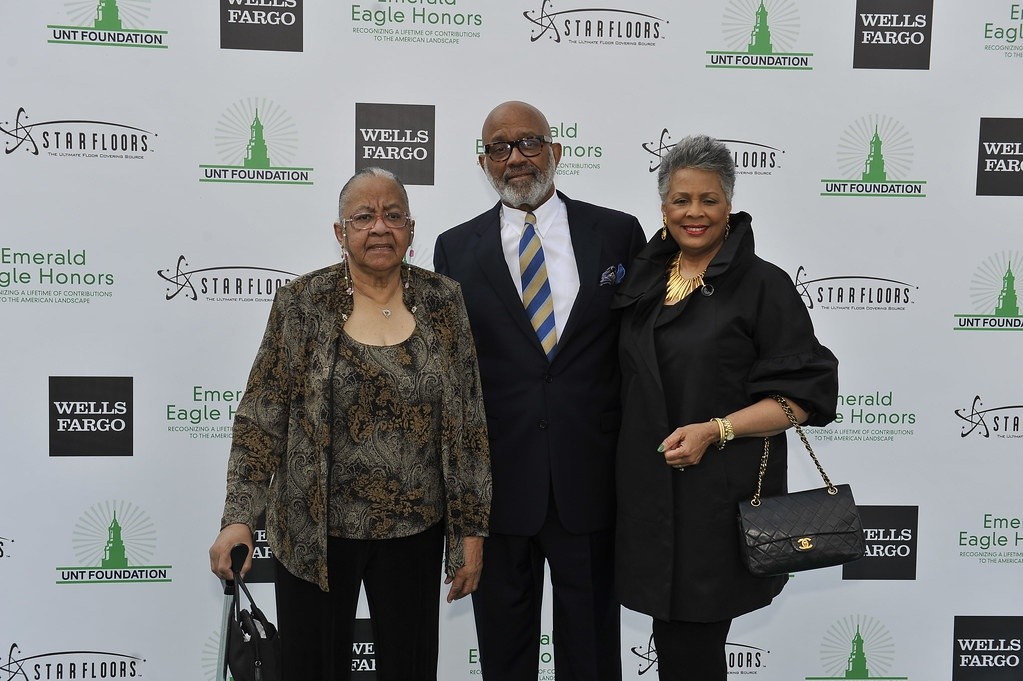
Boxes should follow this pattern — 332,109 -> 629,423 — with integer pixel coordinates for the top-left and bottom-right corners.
677,463 -> 684,470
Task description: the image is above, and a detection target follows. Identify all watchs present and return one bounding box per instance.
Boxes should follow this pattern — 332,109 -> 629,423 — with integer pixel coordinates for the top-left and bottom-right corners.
721,417 -> 736,441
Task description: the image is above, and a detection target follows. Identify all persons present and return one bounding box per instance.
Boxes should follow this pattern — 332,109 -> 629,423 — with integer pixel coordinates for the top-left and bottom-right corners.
434,98 -> 650,680
615,126 -> 839,680
207,167 -> 494,681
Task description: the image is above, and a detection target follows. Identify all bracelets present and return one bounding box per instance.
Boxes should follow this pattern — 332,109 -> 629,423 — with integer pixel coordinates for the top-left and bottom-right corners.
710,418 -> 724,448
717,418 -> 727,451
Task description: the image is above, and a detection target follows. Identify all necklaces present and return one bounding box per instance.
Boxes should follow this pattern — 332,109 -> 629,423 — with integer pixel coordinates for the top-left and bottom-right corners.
350,275 -> 403,319
663,249 -> 708,304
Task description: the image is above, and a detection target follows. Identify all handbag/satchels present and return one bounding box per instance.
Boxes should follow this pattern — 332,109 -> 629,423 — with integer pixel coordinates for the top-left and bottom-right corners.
739,400 -> 866,575
227,571 -> 282,681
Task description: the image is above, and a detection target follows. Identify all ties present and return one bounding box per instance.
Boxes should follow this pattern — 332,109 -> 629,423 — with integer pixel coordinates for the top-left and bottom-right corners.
518,214 -> 556,363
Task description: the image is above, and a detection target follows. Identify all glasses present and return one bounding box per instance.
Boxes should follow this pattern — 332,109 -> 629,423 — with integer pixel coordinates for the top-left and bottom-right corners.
340,211 -> 411,230
484,136 -> 552,162
